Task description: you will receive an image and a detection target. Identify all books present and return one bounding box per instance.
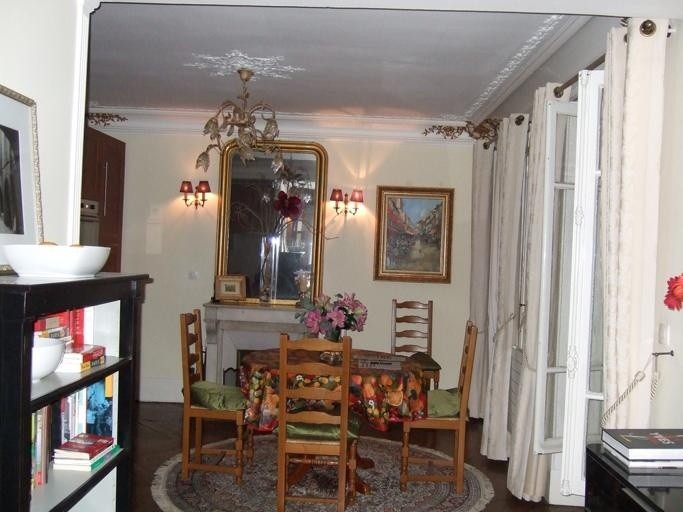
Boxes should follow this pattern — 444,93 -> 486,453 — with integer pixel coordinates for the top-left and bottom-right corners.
27,306 -> 125,490
598,424 -> 683,489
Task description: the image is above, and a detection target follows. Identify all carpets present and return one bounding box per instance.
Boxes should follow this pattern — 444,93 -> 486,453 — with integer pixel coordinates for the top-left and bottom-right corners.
151,430 -> 496,511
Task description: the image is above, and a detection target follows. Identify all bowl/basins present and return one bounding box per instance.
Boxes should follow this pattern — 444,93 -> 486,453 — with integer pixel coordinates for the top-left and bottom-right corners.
30,337 -> 64,386
2,244 -> 111,278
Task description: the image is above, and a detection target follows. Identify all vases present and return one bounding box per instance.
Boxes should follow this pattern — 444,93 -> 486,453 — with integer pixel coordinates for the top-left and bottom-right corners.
254,231 -> 281,306
317,336 -> 343,366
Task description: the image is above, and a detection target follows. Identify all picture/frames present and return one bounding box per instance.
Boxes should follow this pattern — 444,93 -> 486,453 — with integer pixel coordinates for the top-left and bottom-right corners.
0,84 -> 47,277
374,185 -> 456,282
211,273 -> 247,303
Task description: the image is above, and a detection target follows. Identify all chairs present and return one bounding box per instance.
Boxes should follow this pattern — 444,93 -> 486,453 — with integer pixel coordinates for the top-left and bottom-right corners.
278,329 -> 356,510
397,317 -> 483,492
389,296 -> 440,391
178,307 -> 256,488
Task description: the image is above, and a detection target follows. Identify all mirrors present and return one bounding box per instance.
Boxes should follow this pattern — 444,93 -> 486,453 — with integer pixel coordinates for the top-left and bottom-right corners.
213,132 -> 329,307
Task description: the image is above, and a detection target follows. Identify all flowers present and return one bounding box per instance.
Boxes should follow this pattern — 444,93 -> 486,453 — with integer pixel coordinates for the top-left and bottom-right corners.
229,165 -> 332,300
295,292 -> 369,336
662,274 -> 682,313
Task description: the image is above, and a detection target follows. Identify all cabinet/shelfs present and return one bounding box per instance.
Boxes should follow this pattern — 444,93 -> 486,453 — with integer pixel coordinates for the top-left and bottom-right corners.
0,273 -> 155,512
80,123 -> 127,272
279,251 -> 306,297
584,443 -> 683,512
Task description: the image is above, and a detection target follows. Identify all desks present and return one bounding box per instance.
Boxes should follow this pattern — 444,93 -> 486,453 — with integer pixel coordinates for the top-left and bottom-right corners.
237,344 -> 422,495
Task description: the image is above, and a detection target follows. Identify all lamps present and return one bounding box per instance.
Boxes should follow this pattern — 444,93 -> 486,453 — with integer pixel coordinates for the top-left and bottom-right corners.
329,187 -> 366,217
180,177 -> 209,209
189,67 -> 292,175
